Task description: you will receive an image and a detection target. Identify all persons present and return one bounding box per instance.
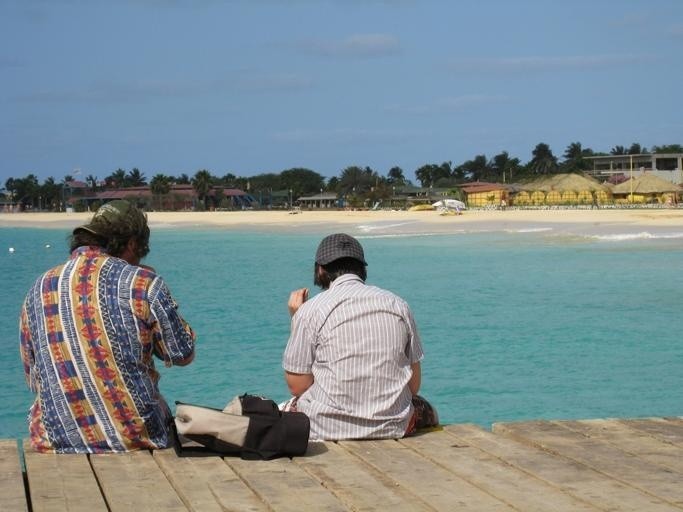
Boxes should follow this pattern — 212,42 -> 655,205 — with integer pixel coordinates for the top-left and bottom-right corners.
19,200 -> 194,456
358,198 -> 509,216
272,230 -> 435,443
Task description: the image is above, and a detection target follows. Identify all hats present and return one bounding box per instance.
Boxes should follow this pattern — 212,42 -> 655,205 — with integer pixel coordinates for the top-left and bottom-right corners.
73,199 -> 150,244
315,233 -> 368,265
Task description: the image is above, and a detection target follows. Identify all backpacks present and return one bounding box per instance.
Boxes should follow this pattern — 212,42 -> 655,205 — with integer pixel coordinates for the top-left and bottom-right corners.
168,393 -> 310,460
